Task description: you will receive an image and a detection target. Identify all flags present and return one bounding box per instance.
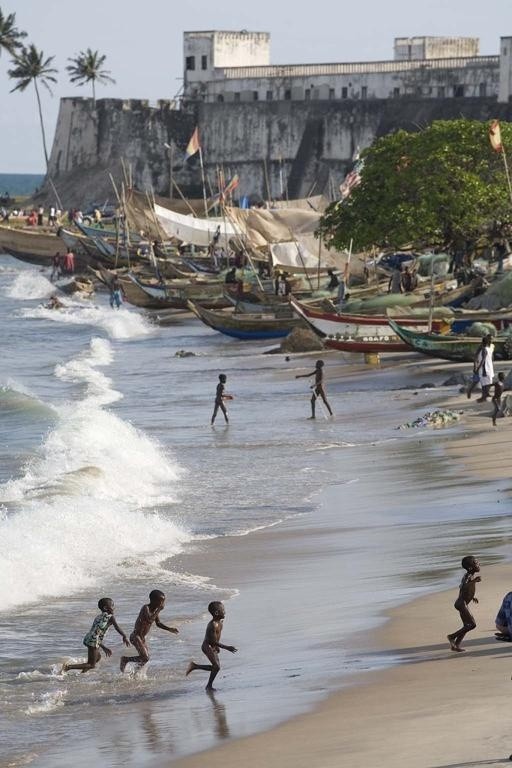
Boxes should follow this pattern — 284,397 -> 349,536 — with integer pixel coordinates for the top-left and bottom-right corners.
181,129 -> 197,161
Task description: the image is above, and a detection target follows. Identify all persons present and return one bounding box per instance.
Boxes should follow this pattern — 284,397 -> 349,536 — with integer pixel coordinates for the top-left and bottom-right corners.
59,598 -> 131,677
295,358 -> 333,420
466,335 -> 494,399
446,556 -> 482,652
185,601 -> 239,692
119,589 -> 180,674
489,369 -> 505,426
210,372 -> 236,426
492,588 -> 511,644
474,336 -> 492,406
